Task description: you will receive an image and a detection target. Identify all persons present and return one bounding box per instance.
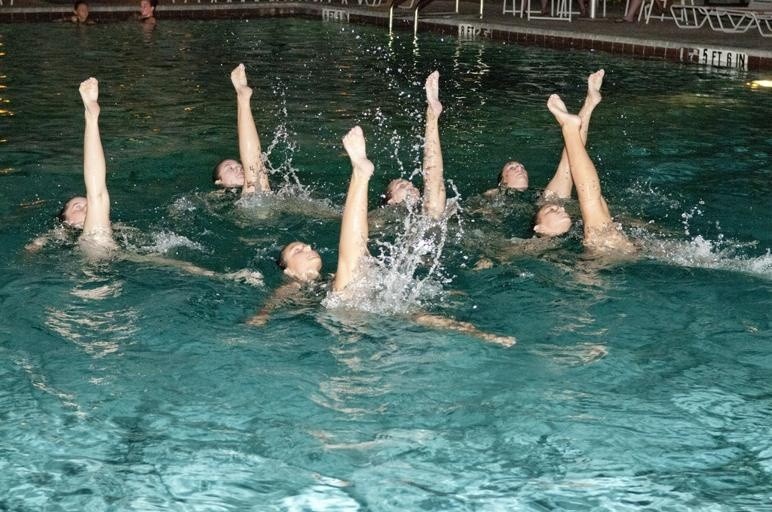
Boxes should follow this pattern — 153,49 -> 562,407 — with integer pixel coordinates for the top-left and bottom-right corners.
212,60 -> 271,192
68,0 -> 96,25
58,76 -> 114,241
499,68 -> 612,237
279,126 -> 374,295
521,0 -> 663,22
381,70 -> 447,217
137,0 -> 157,24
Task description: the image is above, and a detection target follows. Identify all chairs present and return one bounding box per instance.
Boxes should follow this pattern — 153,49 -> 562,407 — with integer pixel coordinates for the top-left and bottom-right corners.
319,0 -> 393,7
624,1 -> 772,38
502,1 -> 606,22
